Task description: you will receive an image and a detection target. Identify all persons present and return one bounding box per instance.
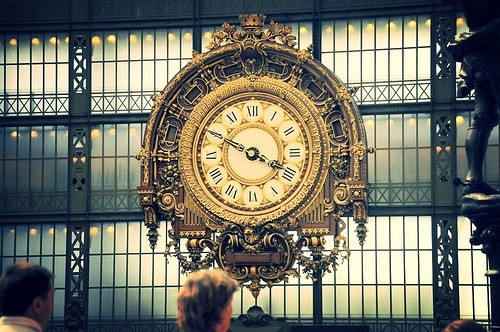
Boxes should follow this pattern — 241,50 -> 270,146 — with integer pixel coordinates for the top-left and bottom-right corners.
176,269 -> 239,332
445,1 -> 500,196
1,262 -> 56,332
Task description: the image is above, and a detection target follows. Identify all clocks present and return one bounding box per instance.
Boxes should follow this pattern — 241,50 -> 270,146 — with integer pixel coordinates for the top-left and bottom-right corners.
132,16 -> 371,305
